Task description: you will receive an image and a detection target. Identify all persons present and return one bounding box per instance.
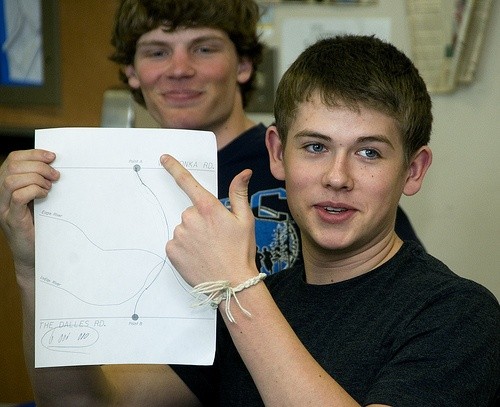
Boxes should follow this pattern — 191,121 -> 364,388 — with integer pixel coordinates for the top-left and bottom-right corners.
1,35 -> 500,407
107,0 -> 419,275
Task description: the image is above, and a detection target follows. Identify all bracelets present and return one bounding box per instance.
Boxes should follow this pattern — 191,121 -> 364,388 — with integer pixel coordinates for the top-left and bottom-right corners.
188,272 -> 265,323
